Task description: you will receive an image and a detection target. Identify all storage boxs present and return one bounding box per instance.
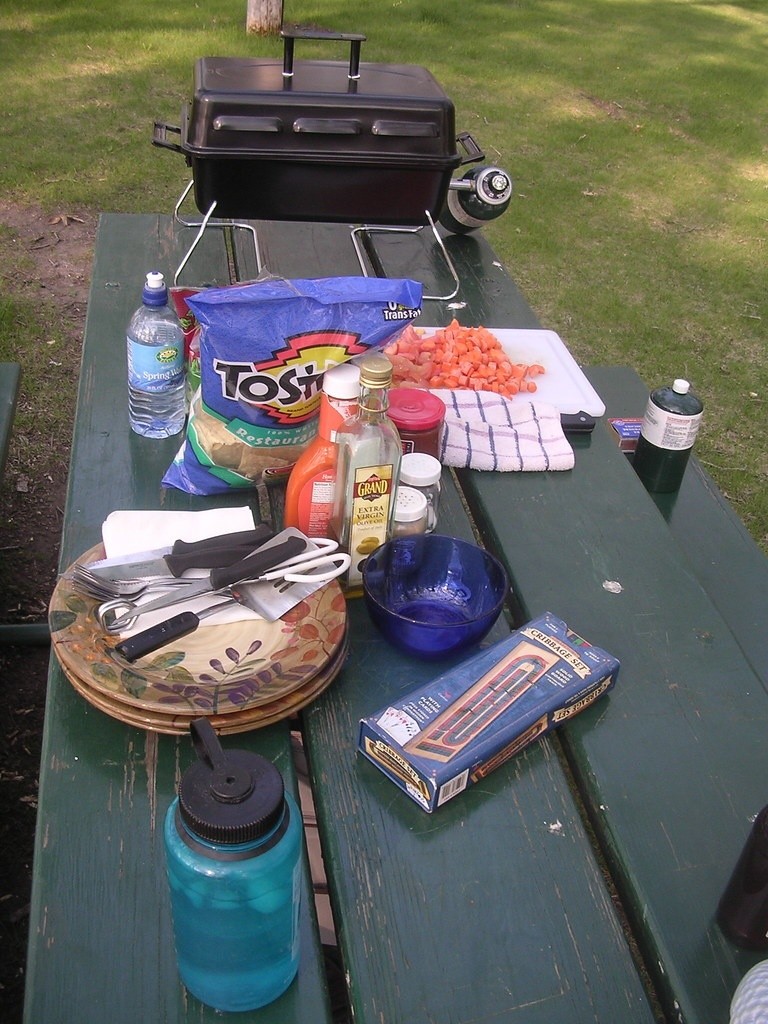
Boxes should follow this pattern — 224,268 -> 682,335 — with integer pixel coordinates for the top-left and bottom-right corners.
356,621 -> 619,814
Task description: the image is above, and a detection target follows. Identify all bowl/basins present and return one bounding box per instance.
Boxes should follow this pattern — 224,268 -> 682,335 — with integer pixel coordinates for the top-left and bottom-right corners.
363,534 -> 508,654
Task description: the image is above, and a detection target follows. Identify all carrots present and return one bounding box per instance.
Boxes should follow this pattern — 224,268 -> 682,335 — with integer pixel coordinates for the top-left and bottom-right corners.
431,319 -> 546,399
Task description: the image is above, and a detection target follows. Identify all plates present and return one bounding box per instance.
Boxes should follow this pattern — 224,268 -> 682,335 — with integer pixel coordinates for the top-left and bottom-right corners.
47,541 -> 350,735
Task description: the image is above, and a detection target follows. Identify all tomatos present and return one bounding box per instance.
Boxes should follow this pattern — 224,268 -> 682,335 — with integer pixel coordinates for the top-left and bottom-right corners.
383,325 -> 442,390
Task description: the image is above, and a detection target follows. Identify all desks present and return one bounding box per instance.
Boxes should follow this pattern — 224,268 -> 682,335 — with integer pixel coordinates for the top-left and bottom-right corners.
20,213 -> 767,1024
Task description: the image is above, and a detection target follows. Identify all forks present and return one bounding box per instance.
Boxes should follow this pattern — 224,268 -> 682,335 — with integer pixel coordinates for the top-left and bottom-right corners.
72,562 -> 207,603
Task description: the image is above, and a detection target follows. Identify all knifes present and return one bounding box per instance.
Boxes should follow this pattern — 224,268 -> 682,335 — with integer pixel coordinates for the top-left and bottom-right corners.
58,525 -> 277,581
112,535 -> 308,624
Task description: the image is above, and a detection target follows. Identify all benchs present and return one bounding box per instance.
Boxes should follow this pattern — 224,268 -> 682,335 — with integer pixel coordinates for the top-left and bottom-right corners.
578,361 -> 767,691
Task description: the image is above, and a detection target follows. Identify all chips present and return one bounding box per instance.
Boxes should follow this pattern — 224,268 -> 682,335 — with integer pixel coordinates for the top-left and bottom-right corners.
192,402 -> 304,479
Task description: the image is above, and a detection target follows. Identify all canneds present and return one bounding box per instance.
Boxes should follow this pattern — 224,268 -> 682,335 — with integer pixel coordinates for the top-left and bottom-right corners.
378,388 -> 447,462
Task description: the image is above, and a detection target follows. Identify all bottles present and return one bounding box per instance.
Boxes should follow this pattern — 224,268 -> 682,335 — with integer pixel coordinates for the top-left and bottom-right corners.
127,271 -> 187,438
384,389 -> 446,460
439,164 -> 512,235
719,805 -> 768,952
164,718 -> 304,1012
285,362 -> 363,541
634,380 -> 704,492
328,357 -> 404,598
389,451 -> 443,538
